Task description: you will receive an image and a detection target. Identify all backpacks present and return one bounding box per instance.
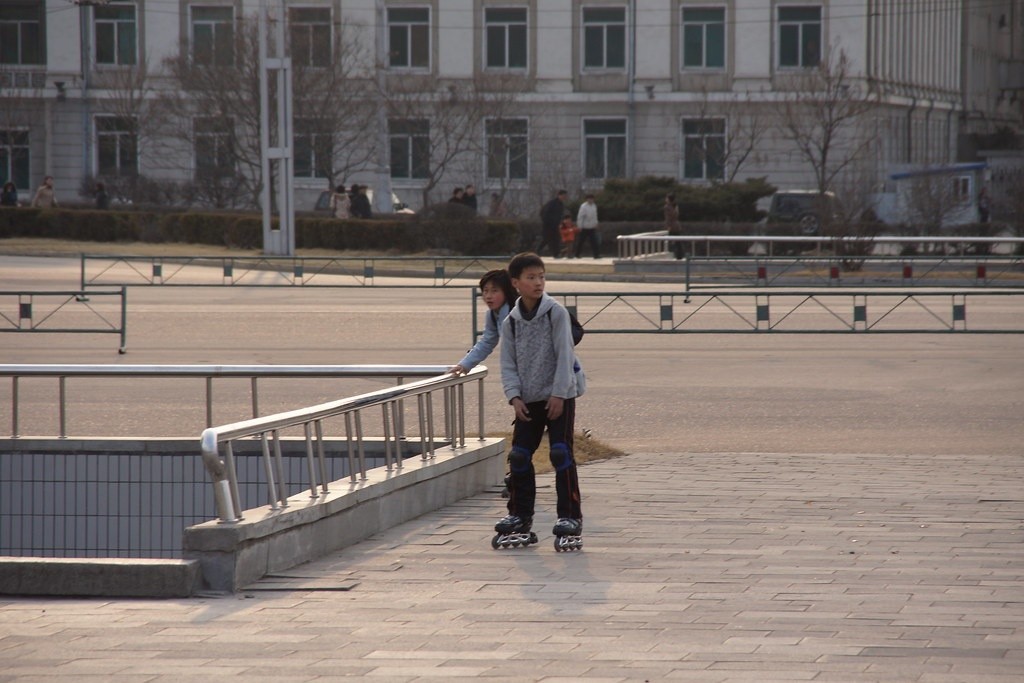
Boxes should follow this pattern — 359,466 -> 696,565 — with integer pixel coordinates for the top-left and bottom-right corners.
509,306 -> 584,346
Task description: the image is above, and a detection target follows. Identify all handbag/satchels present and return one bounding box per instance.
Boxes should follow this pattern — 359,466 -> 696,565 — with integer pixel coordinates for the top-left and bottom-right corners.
331,208 -> 337,217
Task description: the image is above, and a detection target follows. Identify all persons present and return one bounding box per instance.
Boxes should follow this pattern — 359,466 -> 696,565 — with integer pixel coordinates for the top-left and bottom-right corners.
449,188 -> 464,204
662,194 -> 684,259
492,253 -> 583,553
330,185 -> 351,219
556,215 -> 579,258
574,194 -> 603,258
979,187 -> 992,223
489,193 -> 506,216
536,190 -> 569,257
32,177 -> 60,208
463,185 -> 478,209
94,184 -> 106,208
0,183 -> 17,204
449,270 -> 585,498
350,185 -> 370,219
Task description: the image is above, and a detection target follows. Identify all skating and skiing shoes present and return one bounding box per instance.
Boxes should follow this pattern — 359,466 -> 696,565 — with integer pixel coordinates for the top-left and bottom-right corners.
552,517 -> 583,552
491,514 -> 538,549
501,473 -> 513,499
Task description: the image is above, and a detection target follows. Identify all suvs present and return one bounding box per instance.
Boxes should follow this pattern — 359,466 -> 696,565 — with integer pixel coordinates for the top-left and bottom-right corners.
765,190 -> 842,235
